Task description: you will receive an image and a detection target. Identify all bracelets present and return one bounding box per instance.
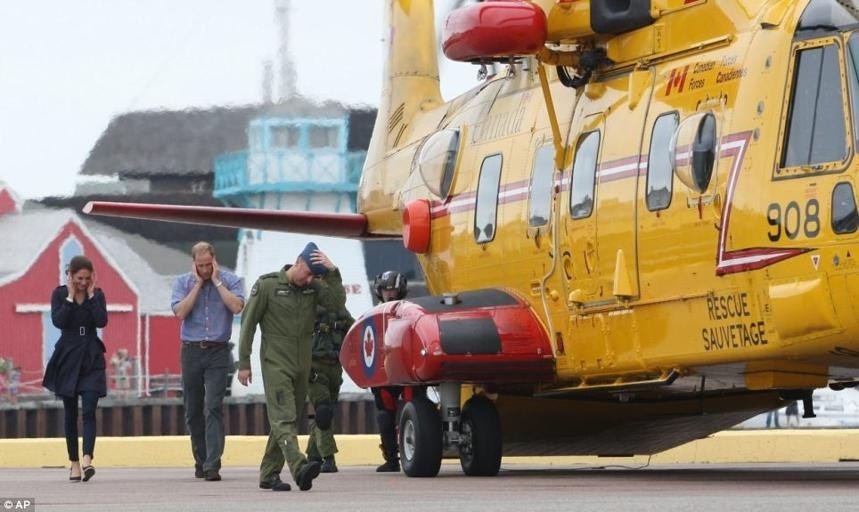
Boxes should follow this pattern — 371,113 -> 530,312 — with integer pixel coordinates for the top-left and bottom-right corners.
215,281 -> 222,287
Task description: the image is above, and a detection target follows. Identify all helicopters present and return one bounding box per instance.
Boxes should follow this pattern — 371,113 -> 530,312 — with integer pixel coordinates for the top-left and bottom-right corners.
76,2 -> 859,480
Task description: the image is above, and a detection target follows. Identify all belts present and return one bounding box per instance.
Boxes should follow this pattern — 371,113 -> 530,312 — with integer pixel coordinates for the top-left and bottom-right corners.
185,340 -> 224,348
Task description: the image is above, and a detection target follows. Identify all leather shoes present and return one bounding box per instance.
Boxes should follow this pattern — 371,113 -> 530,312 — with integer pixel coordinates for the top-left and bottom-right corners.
82,465 -> 94,481
205,470 -> 221,480
196,465 -> 204,478
70,466 -> 81,481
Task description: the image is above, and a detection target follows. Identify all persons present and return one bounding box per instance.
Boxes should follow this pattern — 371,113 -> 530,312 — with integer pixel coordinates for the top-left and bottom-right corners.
8,365 -> 22,405
371,269 -> 428,472
238,243 -> 345,494
111,348 -> 133,389
170,242 -> 245,481
765,409 -> 780,429
785,400 -> 801,427
305,274 -> 357,472
40,256 -> 108,482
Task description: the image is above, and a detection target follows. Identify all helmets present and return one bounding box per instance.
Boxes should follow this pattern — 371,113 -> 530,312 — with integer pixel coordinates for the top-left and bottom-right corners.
374,271 -> 407,301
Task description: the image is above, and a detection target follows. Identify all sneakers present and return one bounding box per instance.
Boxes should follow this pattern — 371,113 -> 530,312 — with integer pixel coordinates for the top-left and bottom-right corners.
296,461 -> 320,490
320,454 -> 338,472
259,480 -> 291,490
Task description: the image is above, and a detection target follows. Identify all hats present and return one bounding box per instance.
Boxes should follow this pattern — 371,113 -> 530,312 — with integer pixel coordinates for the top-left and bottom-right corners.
299,242 -> 327,274
